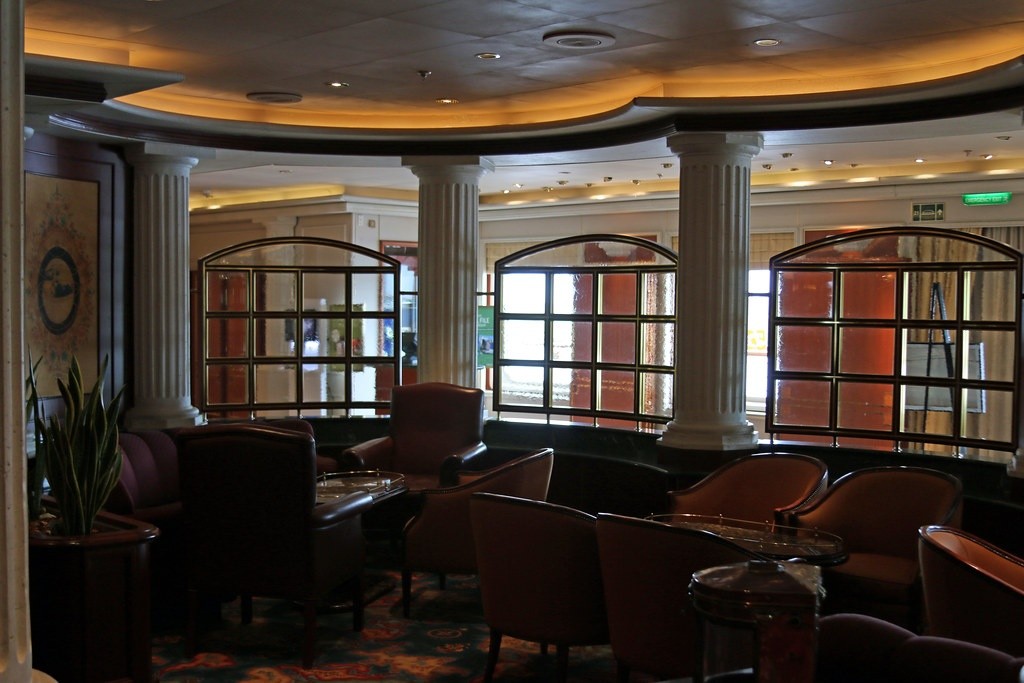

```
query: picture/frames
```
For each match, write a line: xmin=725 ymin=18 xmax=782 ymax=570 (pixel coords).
xmin=283 ymin=308 xmax=316 ymax=340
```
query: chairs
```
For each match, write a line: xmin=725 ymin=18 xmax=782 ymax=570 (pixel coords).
xmin=341 ymin=381 xmax=487 ymax=571
xmin=594 ymin=512 xmax=779 ymax=683
xmin=468 ymin=491 xmax=611 ymax=683
xmin=666 ymin=453 xmax=828 ymax=534
xmin=918 ymin=524 xmax=1024 ymax=657
xmin=401 ymin=448 xmax=553 ymax=618
xmin=793 ymin=467 xmax=962 ymax=620
xmin=177 ymin=424 xmax=371 ymax=669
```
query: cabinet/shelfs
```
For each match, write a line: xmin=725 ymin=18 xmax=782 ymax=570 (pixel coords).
xmin=376 ymin=368 xmax=418 ymax=413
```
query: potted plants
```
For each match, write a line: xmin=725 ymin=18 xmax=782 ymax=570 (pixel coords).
xmin=23 ymin=343 xmax=158 ymax=683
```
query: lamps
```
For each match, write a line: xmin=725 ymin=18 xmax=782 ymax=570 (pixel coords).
xmin=246 ymin=86 xmax=302 ymax=102
xmin=542 ymin=33 xmax=611 ymax=51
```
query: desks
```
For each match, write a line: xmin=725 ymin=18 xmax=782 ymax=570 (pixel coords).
xmin=646 ymin=512 xmax=848 ymax=592
xmin=313 ymin=472 xmax=408 ymax=556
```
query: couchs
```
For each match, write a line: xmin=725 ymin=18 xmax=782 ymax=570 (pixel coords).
xmin=101 ymin=418 xmax=336 ymax=524
xmin=813 ymin=613 xmax=1024 ymax=683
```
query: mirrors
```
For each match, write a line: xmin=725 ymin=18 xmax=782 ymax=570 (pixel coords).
xmin=380 ymin=241 xmax=419 ymax=362
